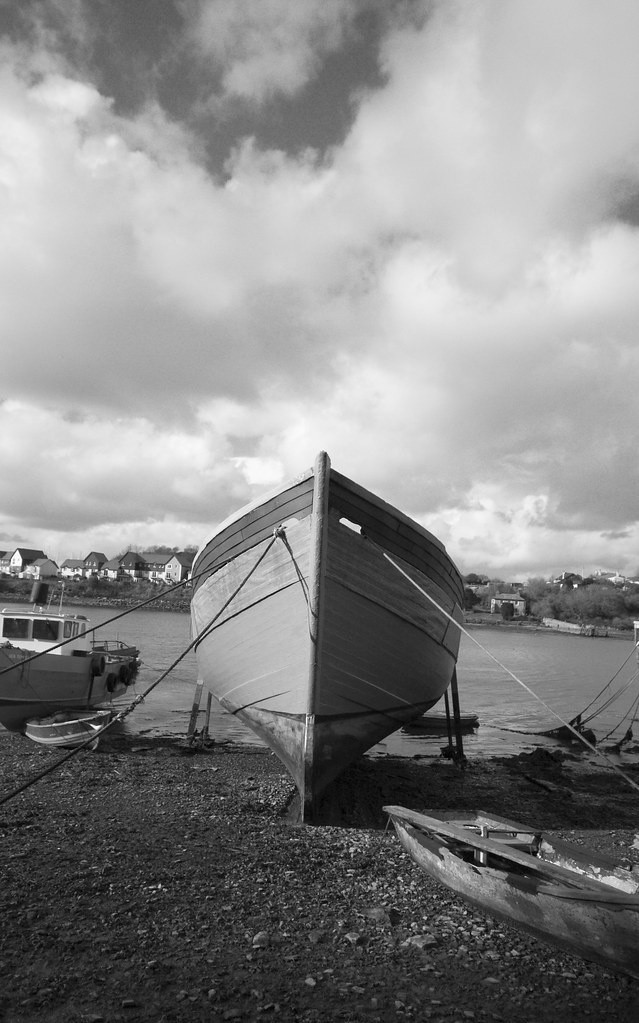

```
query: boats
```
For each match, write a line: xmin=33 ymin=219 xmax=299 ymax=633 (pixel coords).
xmin=0 ymin=581 xmax=141 ymax=731
xmin=402 ymin=713 xmax=480 ymax=732
xmin=389 ymin=806 xmax=639 ymax=982
xmin=25 ymin=709 xmax=111 ymax=750
xmin=189 ymin=452 xmax=465 ymax=822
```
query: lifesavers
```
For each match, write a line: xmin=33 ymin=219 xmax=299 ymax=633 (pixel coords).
xmin=120 ymin=662 xmax=135 ymax=687
xmin=91 ymin=653 xmax=106 ymax=677
xmin=107 ymin=673 xmax=118 ymax=692
